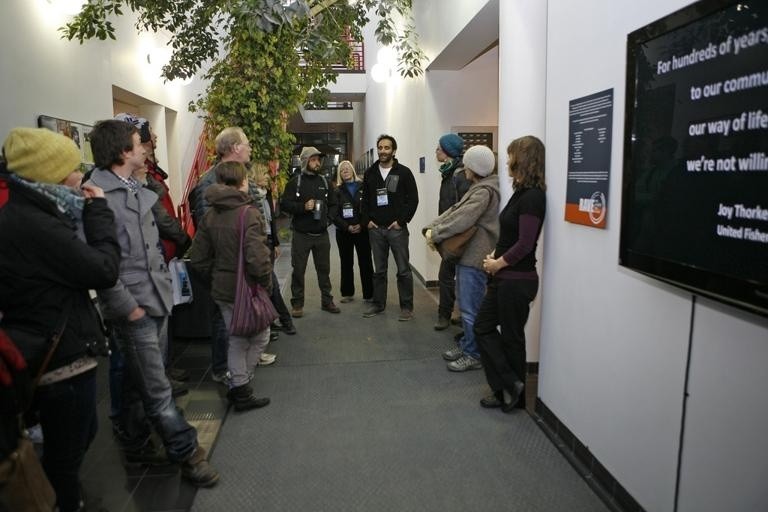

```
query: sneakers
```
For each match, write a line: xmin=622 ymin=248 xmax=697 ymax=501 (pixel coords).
xmin=213 ymin=354 xmax=277 ymax=411
xmin=321 ymin=303 xmax=339 ymax=313
xmin=363 ymin=305 xmax=385 ymax=317
xmin=125 ymin=443 xmax=219 ymax=487
xmin=399 ymin=309 xmax=414 ymax=321
xmin=481 ymin=379 xmax=524 ymax=412
xmin=434 ymin=316 xmax=463 ymax=330
xmin=442 ymin=346 xmax=482 ymax=371
xmin=280 ymin=308 xmax=302 ymax=335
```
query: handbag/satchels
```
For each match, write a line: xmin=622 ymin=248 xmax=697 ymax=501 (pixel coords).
xmin=435 ymin=226 xmax=477 ymax=262
xmin=229 ymin=276 xmax=279 ymax=336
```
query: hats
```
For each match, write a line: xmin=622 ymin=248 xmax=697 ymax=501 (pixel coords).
xmin=463 ymin=145 xmax=495 ymax=177
xmin=439 ymin=134 xmax=464 ymax=157
xmin=115 ymin=112 xmax=150 ymax=143
xmin=5 ymin=127 xmax=82 ymax=184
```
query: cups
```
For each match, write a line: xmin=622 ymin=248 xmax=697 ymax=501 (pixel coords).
xmin=311 ymin=200 xmax=322 ymax=220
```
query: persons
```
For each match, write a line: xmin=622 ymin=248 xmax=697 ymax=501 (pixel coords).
xmin=470 ymin=133 xmax=546 ymax=414
xmin=329 ymin=159 xmax=375 ymax=307
xmin=419 ymin=144 xmax=504 ymax=371
xmin=71 ymin=129 xmax=80 ymax=149
xmin=81 ymin=114 xmax=192 ymax=415
xmin=277 ymin=142 xmax=342 ymax=318
xmin=83 ymin=119 xmax=220 ymax=487
xmin=432 ymin=131 xmax=475 ymax=332
xmin=360 ymin=133 xmax=419 ymax=324
xmin=186 ymin=127 xmax=297 ymax=413
xmin=1 ymin=125 xmax=121 ymax=512
